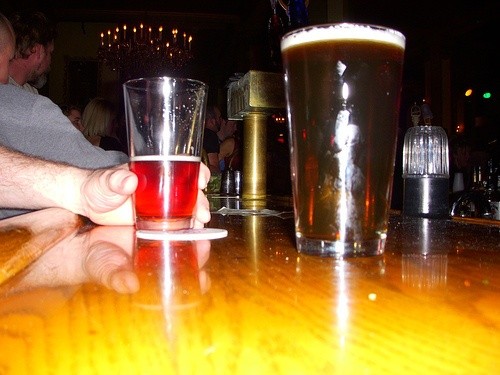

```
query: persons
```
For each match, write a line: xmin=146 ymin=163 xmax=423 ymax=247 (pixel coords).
xmin=0 ymin=10 xmax=238 ymax=229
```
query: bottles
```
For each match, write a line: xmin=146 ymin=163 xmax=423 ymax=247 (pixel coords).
xmin=220 ymin=168 xmax=236 ymax=196
xmin=234 ymin=171 xmax=242 ymax=194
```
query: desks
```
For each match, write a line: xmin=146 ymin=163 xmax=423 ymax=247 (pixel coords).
xmin=0 ymin=192 xmax=500 ymax=374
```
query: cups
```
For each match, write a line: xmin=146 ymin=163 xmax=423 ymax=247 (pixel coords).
xmin=402 ymin=125 xmax=450 ymax=218
xmin=280 ymin=24 xmax=406 ymax=256
xmin=123 ymin=78 xmax=207 ymax=231
